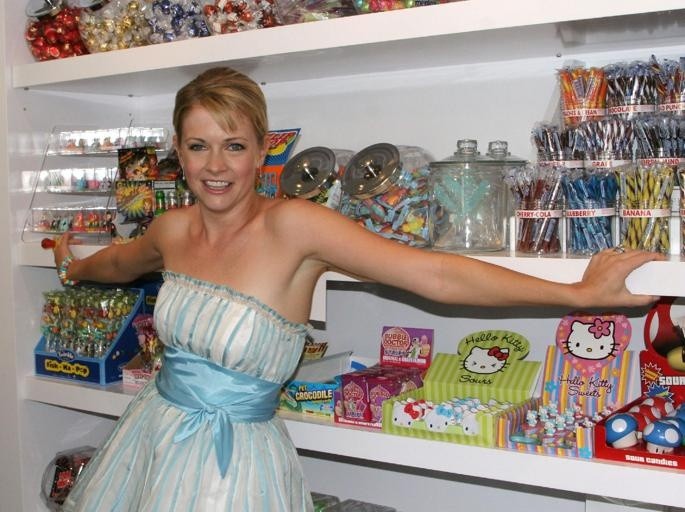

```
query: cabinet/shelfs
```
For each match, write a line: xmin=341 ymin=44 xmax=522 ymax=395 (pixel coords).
xmin=2 ymin=0 xmax=685 ymax=511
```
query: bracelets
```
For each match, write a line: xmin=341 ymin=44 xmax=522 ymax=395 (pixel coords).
xmin=57 ymin=255 xmax=81 ymax=288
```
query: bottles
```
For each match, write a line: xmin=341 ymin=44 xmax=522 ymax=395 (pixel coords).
xmin=426 ymin=136 xmax=534 ymax=252
xmin=333 ymin=142 xmax=457 ymax=252
xmin=276 ymin=145 xmax=354 ymax=216
xmin=23 ymin=0 xmax=449 ymax=62
xmin=151 ymin=190 xmax=194 ymax=222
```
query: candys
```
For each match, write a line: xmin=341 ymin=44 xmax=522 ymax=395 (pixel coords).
xmin=22 ymin=0 xmax=467 ymax=63
xmin=513 ymin=55 xmax=685 ymax=257
xmin=40 ymin=284 xmax=139 ymax=360
xmin=337 ymin=164 xmax=446 ymax=249
xmin=131 ymin=313 xmax=161 ymax=369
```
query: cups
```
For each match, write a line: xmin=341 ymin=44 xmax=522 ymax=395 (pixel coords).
xmin=514 ymin=94 xmax=684 ymax=263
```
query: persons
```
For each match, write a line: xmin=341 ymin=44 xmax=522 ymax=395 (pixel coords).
xmin=51 ymin=64 xmax=672 ymax=510
